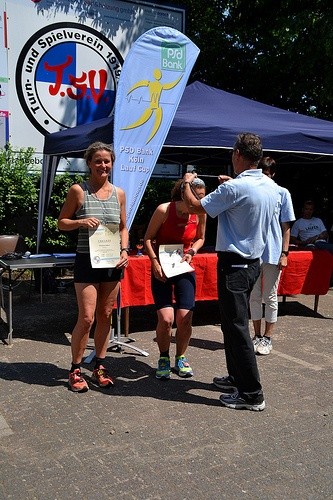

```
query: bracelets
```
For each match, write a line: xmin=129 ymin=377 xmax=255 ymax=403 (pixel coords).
xmin=186 ymin=248 xmax=197 ymax=256
xmin=150 ymin=257 xmax=158 ymax=260
xmin=183 ymin=182 xmax=191 ymax=184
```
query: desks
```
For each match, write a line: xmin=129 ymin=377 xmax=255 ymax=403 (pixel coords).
xmin=113 ymin=248 xmax=333 ymax=337
xmin=1 ymin=257 xmax=121 ymax=345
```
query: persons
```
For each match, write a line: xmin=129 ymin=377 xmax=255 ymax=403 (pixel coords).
xmin=290 ymin=200 xmax=333 ymax=254
xmin=184 ymin=133 xmax=279 ymax=410
xmin=144 ymin=177 xmax=206 ymax=380
xmin=250 ymin=157 xmax=296 ymax=355
xmin=58 ymin=142 xmax=128 ymax=392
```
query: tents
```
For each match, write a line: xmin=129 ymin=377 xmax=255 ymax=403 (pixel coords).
xmin=36 ymin=80 xmax=333 ymax=255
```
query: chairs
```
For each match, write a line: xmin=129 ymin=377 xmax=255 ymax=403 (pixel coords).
xmin=0 ymin=235 xmax=33 ymax=302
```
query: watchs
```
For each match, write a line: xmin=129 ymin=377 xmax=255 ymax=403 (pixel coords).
xmin=281 ymin=250 xmax=288 ymax=257
xmin=119 ymin=248 xmax=129 ymax=255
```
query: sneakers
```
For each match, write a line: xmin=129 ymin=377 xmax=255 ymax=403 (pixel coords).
xmin=68 ymin=368 xmax=90 ymax=393
xmin=257 ymin=337 xmax=273 ymax=355
xmin=213 ymin=375 xmax=238 ymax=389
xmin=174 ymin=354 xmax=193 ymax=378
xmin=252 ymin=336 xmax=263 ymax=352
xmin=220 ymin=391 xmax=265 ymax=411
xmin=91 ymin=365 xmax=114 ymax=386
xmin=155 ymin=356 xmax=171 ymax=379
